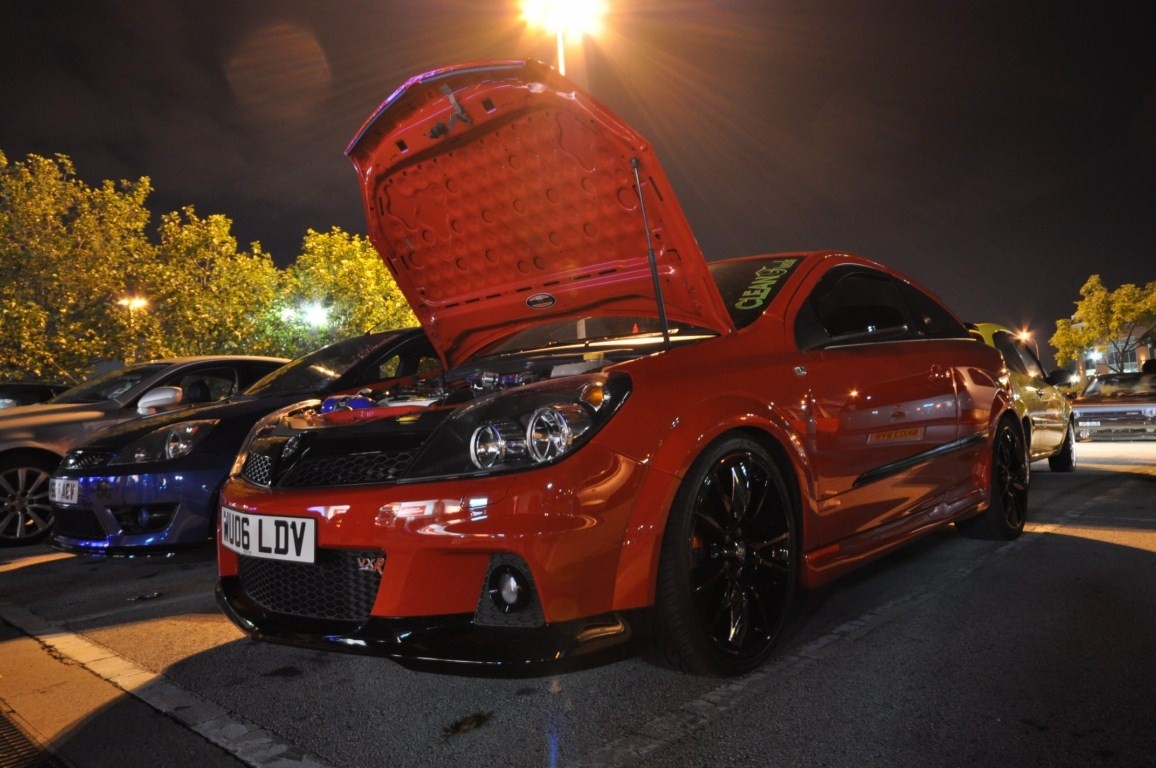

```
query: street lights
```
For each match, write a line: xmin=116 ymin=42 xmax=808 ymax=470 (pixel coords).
xmin=523 ymin=0 xmax=611 ymax=78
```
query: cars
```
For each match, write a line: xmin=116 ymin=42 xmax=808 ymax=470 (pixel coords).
xmin=213 ymin=54 xmax=1031 ymax=678
xmin=0 ymin=354 xmax=345 ymax=547
xmin=1070 ymin=371 xmax=1156 ymax=443
xmin=962 ymin=321 xmax=1077 ymax=474
xmin=42 ymin=324 xmax=445 ymax=561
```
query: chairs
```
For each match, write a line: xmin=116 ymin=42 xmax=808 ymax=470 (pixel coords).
xmin=187 ymin=380 xmax=211 ymax=404
